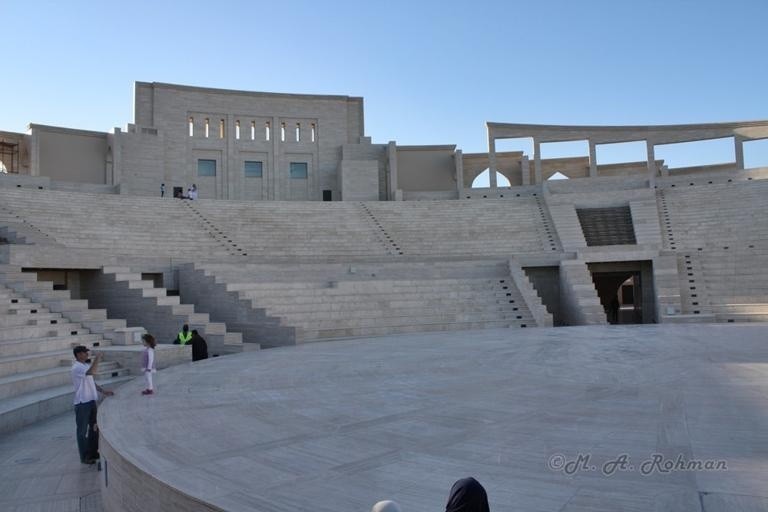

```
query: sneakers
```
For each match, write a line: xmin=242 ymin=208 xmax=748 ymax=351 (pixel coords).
xmin=80 ymin=451 xmax=101 ymax=464
xmin=142 ymin=389 xmax=154 ymax=395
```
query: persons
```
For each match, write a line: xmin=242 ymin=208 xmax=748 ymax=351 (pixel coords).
xmin=184 ymin=330 xmax=209 ymax=362
xmin=177 ymin=190 xmax=186 ymax=199
xmin=187 ymin=188 xmax=191 ymax=198
xmin=445 ymin=476 xmax=489 ymax=511
xmin=172 ymin=324 xmax=191 ymax=345
xmin=141 ymin=334 xmax=155 ymax=394
xmin=160 ymin=183 xmax=165 ymax=197
xmin=70 ymin=344 xmax=117 ymax=467
xmin=190 ymin=183 xmax=197 ymax=200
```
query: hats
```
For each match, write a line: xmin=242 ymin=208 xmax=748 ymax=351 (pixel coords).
xmin=73 ymin=345 xmax=90 ymax=354
